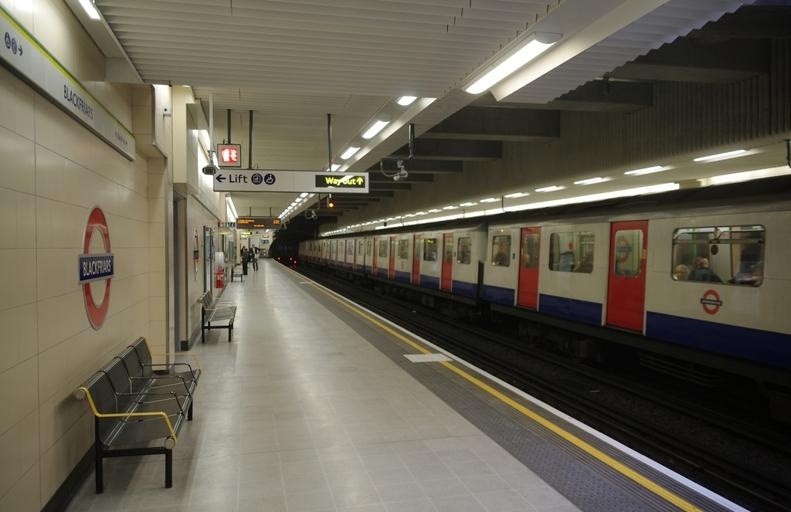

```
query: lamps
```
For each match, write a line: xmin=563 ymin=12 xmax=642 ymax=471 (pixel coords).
xmin=395 ymin=96 xmax=418 ymax=105
xmin=318 ymin=194 xmax=502 ymax=236
xmin=505 ymin=177 xmax=679 ymax=212
xmin=279 ymin=164 xmax=340 ymax=223
xmin=340 ymin=146 xmax=360 ymax=159
xmin=695 ymin=150 xmax=749 ymax=165
xmin=461 ymin=31 xmax=563 ymax=95
xmin=362 ymin=119 xmax=389 ymax=139
xmin=627 ymin=165 xmax=671 ymax=178
xmin=700 ymin=165 xmax=790 ymax=187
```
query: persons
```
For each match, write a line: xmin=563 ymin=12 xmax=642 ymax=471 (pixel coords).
xmin=574 ymin=253 xmax=594 ymax=273
xmin=754 ymin=260 xmax=764 ymax=287
xmin=524 ymin=252 xmax=534 ymax=269
xmin=494 ymin=251 xmax=509 ymax=267
xmin=674 ymin=264 xmax=690 ymax=280
xmin=691 ymin=257 xmax=724 ymax=283
xmin=240 ymin=245 xmax=245 ymax=257
xmin=250 ymin=244 xmax=260 ymax=272
xmin=242 ymin=248 xmax=249 ymax=275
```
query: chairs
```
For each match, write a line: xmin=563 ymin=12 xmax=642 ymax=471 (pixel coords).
xmin=75 ymin=263 xmax=244 ymax=494
